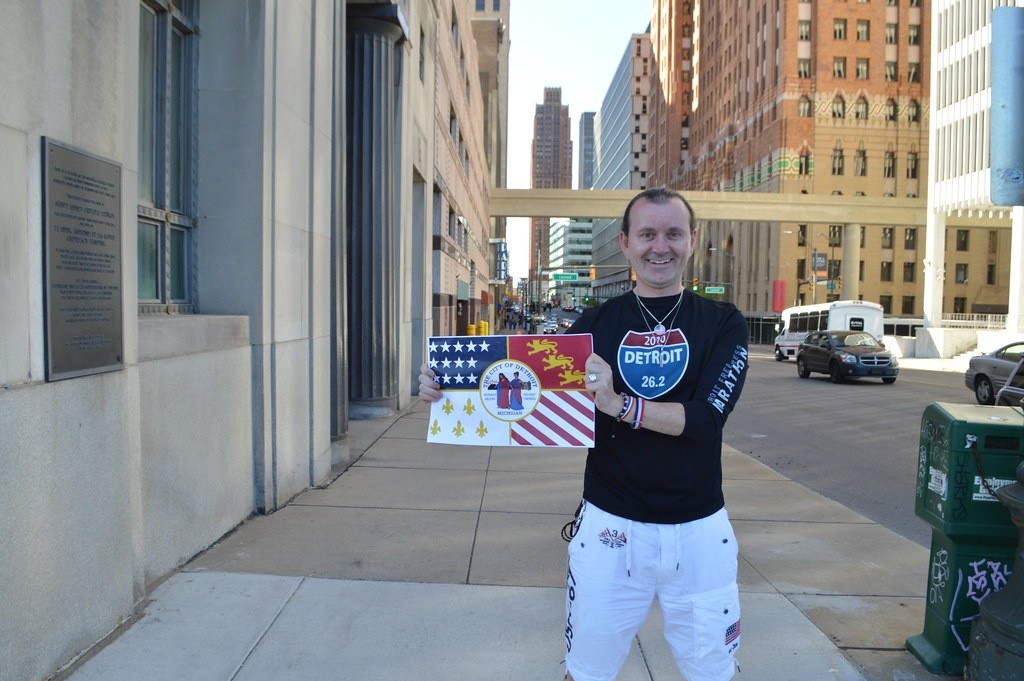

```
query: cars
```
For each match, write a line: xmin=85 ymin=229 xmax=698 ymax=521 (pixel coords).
xmin=964 ymin=341 xmax=1024 ymax=405
xmin=561 ymin=318 xmax=575 ymax=328
xmin=796 ymin=330 xmax=900 ymax=384
xmin=534 ymin=313 xmax=560 ymax=334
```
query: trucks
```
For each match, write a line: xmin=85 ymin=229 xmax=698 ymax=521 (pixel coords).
xmin=773 ymin=300 xmax=884 ymax=362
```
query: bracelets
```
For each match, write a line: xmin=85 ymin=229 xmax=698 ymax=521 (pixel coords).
xmin=630 ymin=397 xmax=645 ymax=430
xmin=616 ymin=391 xmax=634 ymax=423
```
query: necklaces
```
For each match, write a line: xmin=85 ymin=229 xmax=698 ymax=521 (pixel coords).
xmin=636 ymin=289 xmax=683 ymax=368
xmin=633 ymin=289 xmax=683 ymax=336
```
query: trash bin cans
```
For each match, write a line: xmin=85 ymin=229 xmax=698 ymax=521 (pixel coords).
xmin=905 ymin=401 xmax=1024 ymax=675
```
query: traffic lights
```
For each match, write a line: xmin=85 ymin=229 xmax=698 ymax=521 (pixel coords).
xmin=692 ymin=278 xmax=699 ymax=291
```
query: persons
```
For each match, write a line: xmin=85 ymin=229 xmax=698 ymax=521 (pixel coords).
xmin=417 ymin=187 xmax=749 ymax=681
xmin=505 ymin=313 xmax=522 ymax=331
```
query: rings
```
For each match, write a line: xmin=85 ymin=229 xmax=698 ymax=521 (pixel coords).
xmin=589 ymin=373 xmax=597 ymax=383
